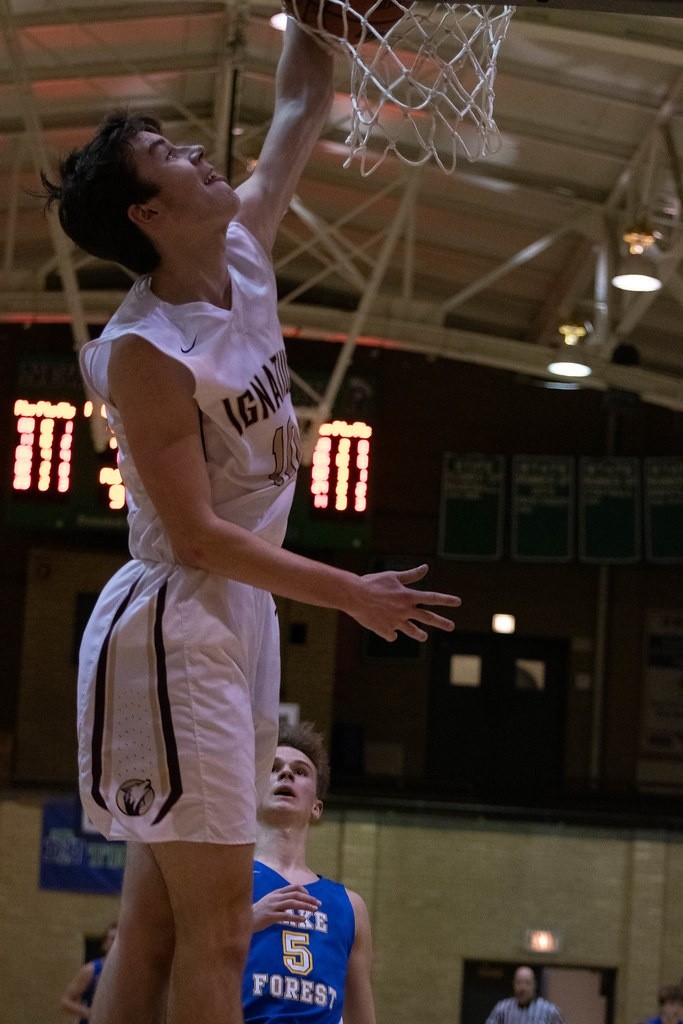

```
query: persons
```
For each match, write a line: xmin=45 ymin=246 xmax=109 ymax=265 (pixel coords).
xmin=485 ymin=966 xmax=565 ymax=1024
xmin=640 ymin=984 xmax=683 ymax=1024
xmin=62 ymin=922 xmax=117 ymax=1023
xmin=36 ymin=19 xmax=461 ymax=1024
xmin=242 ymin=723 xmax=377 ymax=1024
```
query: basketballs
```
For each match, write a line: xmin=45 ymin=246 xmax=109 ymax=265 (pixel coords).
xmin=286 ymin=0 xmax=412 ymax=46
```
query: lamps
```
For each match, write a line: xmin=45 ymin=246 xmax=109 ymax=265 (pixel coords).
xmin=623 ymin=205 xmax=655 ymax=255
xmin=558 ymin=304 xmax=594 ymax=346
xmin=610 ymin=254 xmax=662 ymax=292
xmin=547 ymin=341 xmax=592 ymax=378
